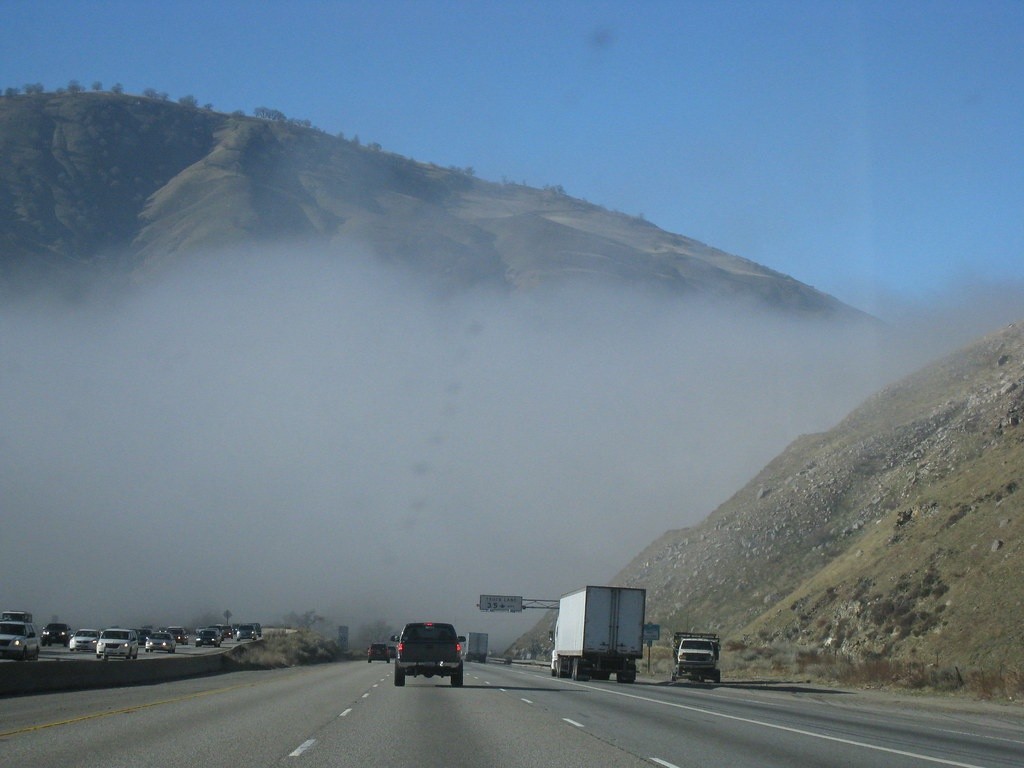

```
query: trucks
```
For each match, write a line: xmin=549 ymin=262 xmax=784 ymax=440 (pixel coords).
xmin=548 ymin=586 xmax=646 ymax=683
xmin=466 ymin=632 xmax=488 ymax=664
xmin=671 ymin=632 xmax=721 ymax=683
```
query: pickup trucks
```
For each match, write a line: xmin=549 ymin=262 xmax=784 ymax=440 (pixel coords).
xmin=390 ymin=622 xmax=466 ymax=688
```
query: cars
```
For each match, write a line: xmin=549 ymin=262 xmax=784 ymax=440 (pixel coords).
xmin=167 ymin=627 xmax=189 ymax=645
xmin=96 ymin=629 xmax=138 ymax=659
xmin=367 ymin=643 xmax=390 ymax=663
xmin=40 ymin=623 xmax=73 ymax=646
xmin=388 ymin=646 xmax=396 ymax=658
xmin=146 ymin=633 xmax=176 ymax=653
xmin=131 ymin=628 xmax=153 ymax=645
xmin=69 ymin=629 xmax=100 ymax=651
xmin=195 ymin=630 xmax=222 ymax=647
xmin=249 ymin=623 xmax=263 ymax=637
xmin=234 ymin=625 xmax=257 ymax=641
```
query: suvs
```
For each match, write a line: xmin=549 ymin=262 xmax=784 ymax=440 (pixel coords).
xmin=0 ymin=619 xmax=41 ymax=661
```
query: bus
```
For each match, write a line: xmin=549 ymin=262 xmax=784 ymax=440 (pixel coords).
xmin=210 ymin=625 xmax=232 ymax=638
xmin=2 ymin=610 xmax=33 ymax=623
